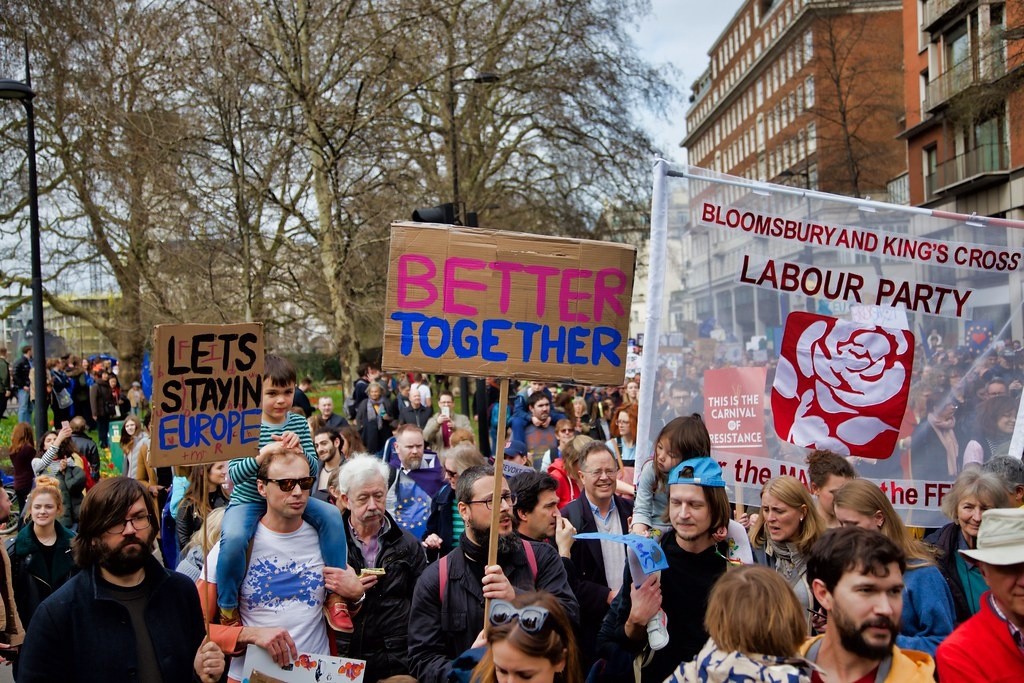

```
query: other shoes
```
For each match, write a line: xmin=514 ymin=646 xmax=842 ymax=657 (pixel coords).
xmin=323 ymin=598 xmax=354 ymax=634
xmin=220 ymin=610 xmax=246 ymax=657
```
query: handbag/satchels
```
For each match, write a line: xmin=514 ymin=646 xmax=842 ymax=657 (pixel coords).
xmin=55 ymin=387 xmax=74 ymax=409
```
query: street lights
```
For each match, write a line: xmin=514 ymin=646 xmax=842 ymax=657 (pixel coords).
xmin=688 ymin=229 xmax=712 ymax=319
xmin=776 ymin=169 xmax=813 ymax=266
xmin=0 ymin=76 xmax=50 ymax=458
xmin=447 ymin=68 xmax=502 ymax=225
xmin=668 ymin=235 xmax=685 ymax=287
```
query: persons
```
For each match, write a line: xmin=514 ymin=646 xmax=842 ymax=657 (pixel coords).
xmin=0 ymin=334 xmax=1024 ymax=683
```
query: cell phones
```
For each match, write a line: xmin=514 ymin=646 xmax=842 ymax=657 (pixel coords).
xmin=807 ymin=608 xmax=827 ymax=619
xmin=61 ymin=420 xmax=70 ymax=429
xmin=442 ymin=407 xmax=450 ymax=418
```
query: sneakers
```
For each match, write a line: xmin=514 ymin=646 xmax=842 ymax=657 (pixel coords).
xmin=647 ymin=608 xmax=670 ymax=650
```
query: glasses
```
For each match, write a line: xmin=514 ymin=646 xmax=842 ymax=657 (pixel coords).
xmin=559 ymin=428 xmax=575 ymax=433
xmin=263 ymin=476 xmax=316 ymax=492
xmin=444 ymin=466 xmax=457 ymax=478
xmin=582 ymin=470 xmax=617 ymax=477
xmin=107 ymin=514 xmax=152 ymax=534
xmin=464 ymin=493 xmax=518 ymax=510
xmin=987 ymin=392 xmax=1005 ymax=398
xmin=489 ymin=599 xmax=569 ymax=645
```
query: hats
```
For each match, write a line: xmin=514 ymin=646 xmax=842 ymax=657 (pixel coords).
xmin=958 ymin=508 xmax=1024 ymax=565
xmin=1000 ymin=347 xmax=1017 ymax=358
xmin=668 ymin=457 xmax=725 ymax=487
xmin=504 ymin=440 xmax=527 ymax=457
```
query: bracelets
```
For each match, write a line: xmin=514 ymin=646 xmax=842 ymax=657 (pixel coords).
xmin=347 ymin=592 xmax=366 ymax=612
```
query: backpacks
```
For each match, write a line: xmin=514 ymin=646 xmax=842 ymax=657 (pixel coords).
xmin=344 ymin=380 xmax=368 ymax=420
